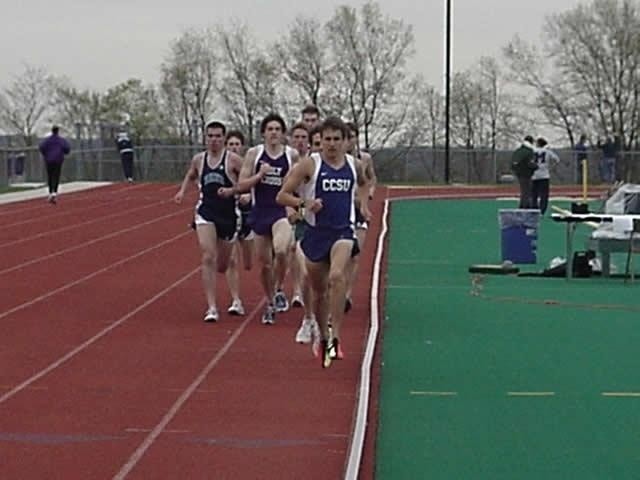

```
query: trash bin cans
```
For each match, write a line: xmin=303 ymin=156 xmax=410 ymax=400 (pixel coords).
xmin=497 ymin=208 xmax=541 ymax=264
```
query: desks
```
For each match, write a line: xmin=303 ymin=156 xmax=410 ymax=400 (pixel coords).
xmin=552 ymin=211 xmax=640 ymax=280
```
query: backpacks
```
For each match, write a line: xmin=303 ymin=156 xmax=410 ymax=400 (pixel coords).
xmin=512 ymin=148 xmax=530 ymax=175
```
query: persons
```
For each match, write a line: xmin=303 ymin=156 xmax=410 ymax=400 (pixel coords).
xmin=597 ymin=138 xmax=620 ymax=185
xmin=575 ymin=134 xmax=586 ymax=184
xmin=38 ymin=125 xmax=72 ymax=202
xmin=511 ymin=136 xmax=537 ymax=208
xmin=114 ymin=126 xmax=135 ymax=184
xmin=521 ymin=139 xmax=560 ymax=215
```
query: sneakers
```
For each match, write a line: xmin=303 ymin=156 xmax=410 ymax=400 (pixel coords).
xmin=343 ymin=291 xmax=352 ymax=313
xmin=295 ymin=314 xmax=344 ymax=369
xmin=227 ymin=297 xmax=248 ymax=315
xmin=272 ymin=288 xmax=288 ymax=313
xmin=204 ymin=307 xmax=220 ymax=323
xmin=260 ymin=303 xmax=276 ymax=326
xmin=47 ymin=192 xmax=57 ymax=205
xmin=291 ymin=290 xmax=304 ymax=307
xmin=127 ymin=176 xmax=136 ymax=184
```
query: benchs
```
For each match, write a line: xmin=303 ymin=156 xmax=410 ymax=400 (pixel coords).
xmin=584 ymin=234 xmax=640 ymax=278
